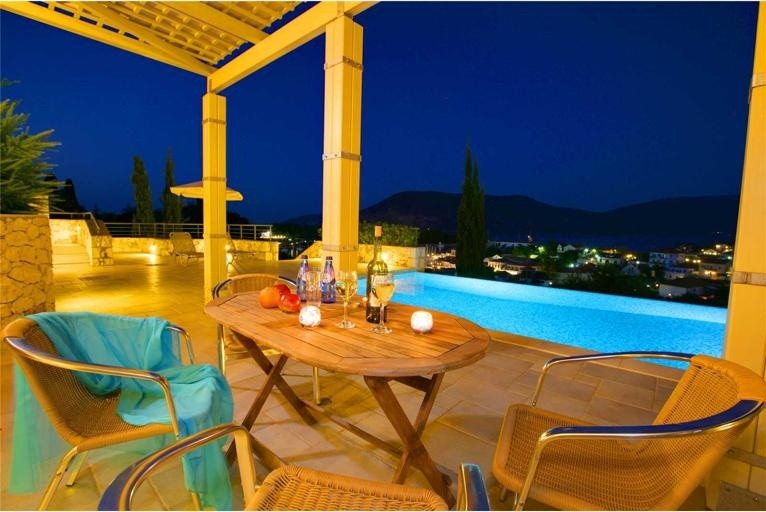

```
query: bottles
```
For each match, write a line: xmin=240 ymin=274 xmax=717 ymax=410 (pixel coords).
xmin=319 ymin=255 xmax=336 ymax=304
xmin=296 ymin=253 xmax=310 ymax=302
xmin=366 ymin=224 xmax=388 ymax=323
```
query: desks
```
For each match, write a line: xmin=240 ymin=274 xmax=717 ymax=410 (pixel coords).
xmin=191 ymin=249 xmax=255 ymax=275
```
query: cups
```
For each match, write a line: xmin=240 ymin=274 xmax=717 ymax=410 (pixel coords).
xmin=305 ymin=269 xmax=323 ymax=308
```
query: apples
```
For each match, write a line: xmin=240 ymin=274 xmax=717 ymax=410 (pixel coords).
xmin=278 ymin=294 xmax=301 ymax=312
xmin=272 ymin=284 xmax=290 ymax=297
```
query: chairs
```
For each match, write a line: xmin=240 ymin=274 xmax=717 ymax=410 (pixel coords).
xmin=491 ymin=350 xmax=766 ymax=511
xmin=99 ymin=422 xmax=490 ymax=511
xmin=170 ymin=232 xmax=198 ymax=265
xmin=211 ymin=273 xmax=322 ymax=407
xmin=1 ymin=312 xmax=234 ymax=511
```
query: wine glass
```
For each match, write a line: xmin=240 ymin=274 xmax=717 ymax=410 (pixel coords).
xmin=333 ymin=269 xmax=358 ymax=330
xmin=369 ymin=271 xmax=396 ymax=334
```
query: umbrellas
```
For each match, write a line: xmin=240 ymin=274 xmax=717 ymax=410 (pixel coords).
xmin=170 ymin=179 xmax=244 ymax=203
